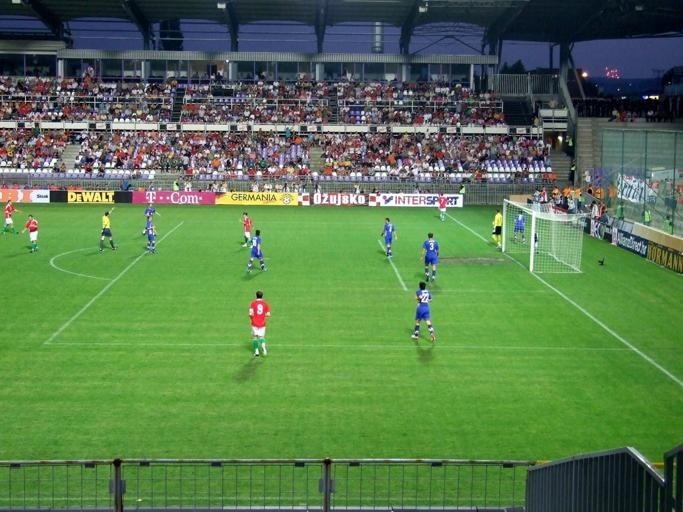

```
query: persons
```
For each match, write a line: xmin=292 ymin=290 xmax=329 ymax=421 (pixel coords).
xmin=240 ymin=212 xmax=254 ymax=249
xmin=411 ymin=282 xmax=436 ymax=344
xmin=142 ymin=223 xmax=158 ymax=254
xmin=1 ymin=69 xmax=682 ymax=234
xmin=246 ymin=229 xmax=267 ymax=272
xmin=99 ymin=207 xmax=118 ymax=251
xmin=21 ymin=214 xmax=39 ymax=254
xmin=380 ymin=217 xmax=397 ymax=258
xmin=513 ymin=210 xmax=527 ymax=244
xmin=420 ymin=232 xmax=439 ymax=284
xmin=248 ymin=291 xmax=271 ymax=358
xmin=491 ymin=208 xmax=503 ymax=249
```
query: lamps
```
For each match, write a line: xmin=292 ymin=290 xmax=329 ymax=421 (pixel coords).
xmin=418 ymin=1 xmax=431 ymax=17
xmin=216 ymin=1 xmax=228 ymax=12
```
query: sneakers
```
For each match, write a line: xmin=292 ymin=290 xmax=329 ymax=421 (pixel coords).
xmin=389 ymin=252 xmax=392 ymax=257
xmin=254 ymin=350 xmax=260 ymax=356
xmin=262 ymin=266 xmax=266 ymax=271
xmin=260 ymin=346 xmax=266 ymax=356
xmin=432 ymin=275 xmax=435 ymax=281
xmin=431 ymin=333 xmax=434 ymax=342
xmin=387 ymin=253 xmax=389 ymax=257
xmin=426 ymin=272 xmax=430 ymax=282
xmin=410 ymin=334 xmax=418 ymax=338
xmin=247 ymin=268 xmax=250 ymax=272
